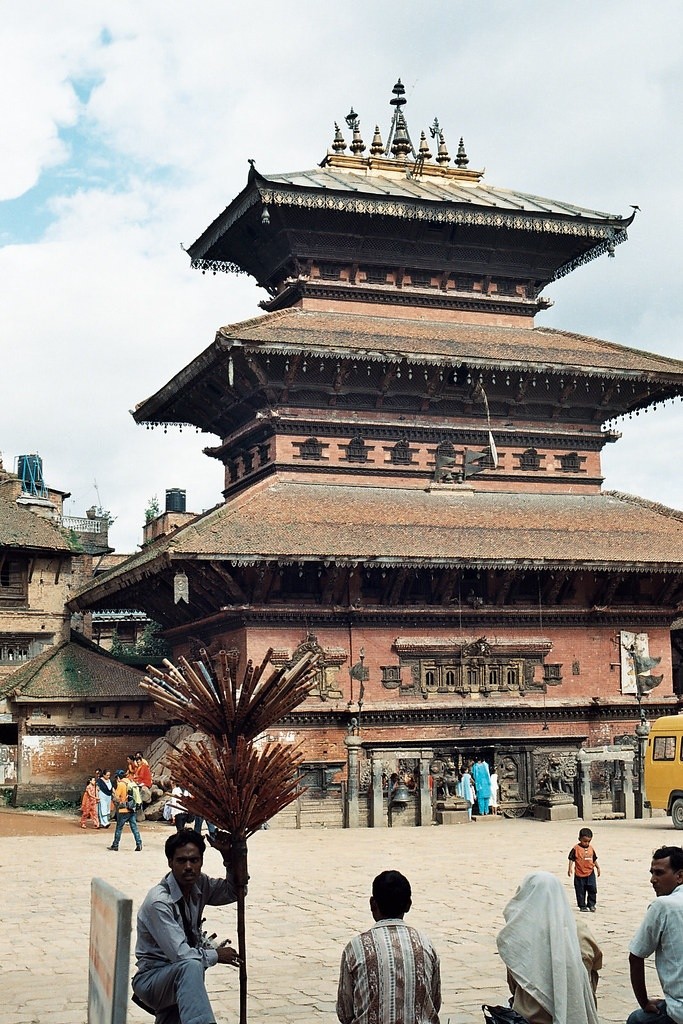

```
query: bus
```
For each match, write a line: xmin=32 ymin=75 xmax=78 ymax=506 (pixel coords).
xmin=642 ymin=714 xmax=682 ymax=830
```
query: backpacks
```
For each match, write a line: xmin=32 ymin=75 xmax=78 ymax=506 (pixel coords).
xmin=118 ymin=778 xmax=143 ymax=813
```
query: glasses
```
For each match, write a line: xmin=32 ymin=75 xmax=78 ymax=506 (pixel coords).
xmin=96 ymin=772 xmax=99 ymax=775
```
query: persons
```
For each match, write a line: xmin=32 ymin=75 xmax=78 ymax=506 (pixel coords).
xmin=105 ymin=770 xmax=144 ymax=851
xmin=336 ymin=870 xmax=441 ymax=1023
xmin=77 ymin=749 xmax=217 ymax=837
xmin=496 ymin=871 xmax=602 ymax=1023
xmin=624 ymin=846 xmax=683 ymax=1023
xmin=566 ymin=828 xmax=603 ymax=910
xmin=130 ymin=828 xmax=244 ymax=1023
xmin=458 ymin=756 xmax=499 ymax=817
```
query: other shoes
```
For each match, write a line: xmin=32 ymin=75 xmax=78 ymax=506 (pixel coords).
xmin=589 ymin=906 xmax=596 ymax=912
xmin=580 ymin=907 xmax=588 ymax=912
xmin=107 ymin=846 xmax=118 ymax=851
xmin=135 ymin=846 xmax=142 ymax=851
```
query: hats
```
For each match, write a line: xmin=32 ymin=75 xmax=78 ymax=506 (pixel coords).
xmin=113 ymin=769 xmax=124 ymax=778
